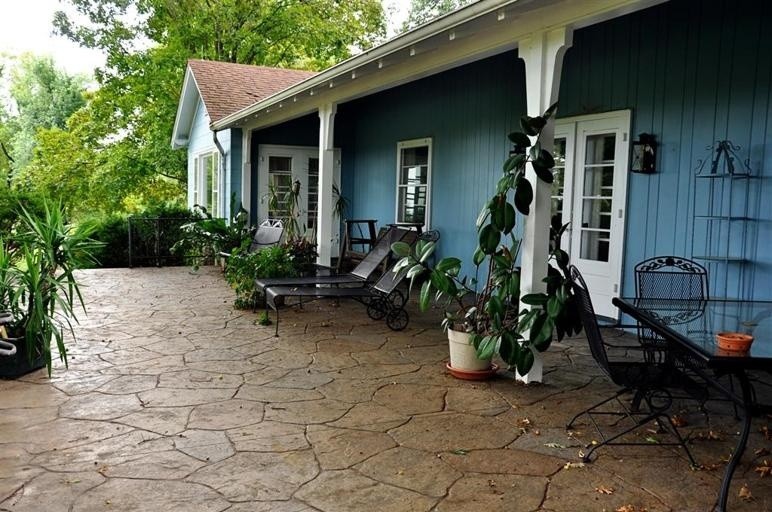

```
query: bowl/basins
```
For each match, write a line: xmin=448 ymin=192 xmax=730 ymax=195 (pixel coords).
xmin=716 ymin=332 xmax=754 ymax=352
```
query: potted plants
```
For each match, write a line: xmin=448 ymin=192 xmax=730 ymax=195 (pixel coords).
xmin=393 ymin=102 xmax=583 ymax=379
xmin=0 ymin=186 xmax=109 ymax=381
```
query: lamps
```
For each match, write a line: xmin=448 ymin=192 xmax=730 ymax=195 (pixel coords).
xmin=628 ymin=129 xmax=658 ymax=176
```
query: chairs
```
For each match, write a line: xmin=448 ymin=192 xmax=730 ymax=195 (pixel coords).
xmin=219 ymin=221 xmax=441 ymax=338
xmin=566 ymin=256 xmax=743 ymax=469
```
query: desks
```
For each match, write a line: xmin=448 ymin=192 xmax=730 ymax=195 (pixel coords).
xmin=613 ymin=296 xmax=772 ymax=512
